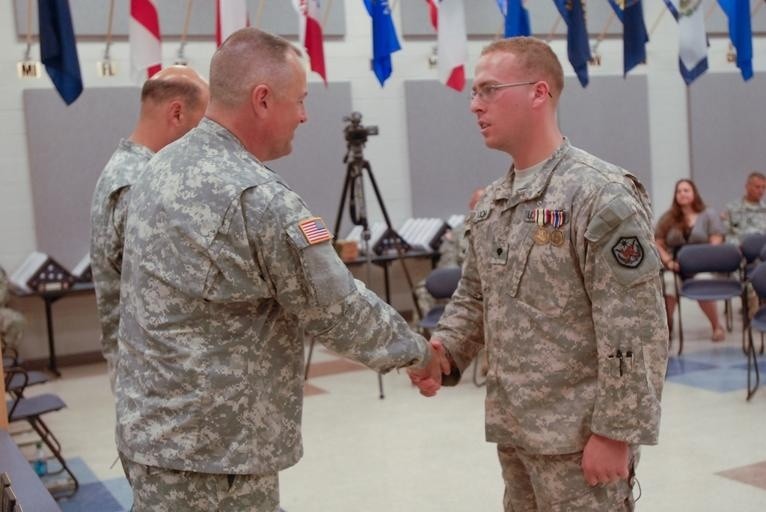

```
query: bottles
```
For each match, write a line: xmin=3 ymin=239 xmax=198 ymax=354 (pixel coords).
xmin=32 ymin=442 xmax=46 ymax=475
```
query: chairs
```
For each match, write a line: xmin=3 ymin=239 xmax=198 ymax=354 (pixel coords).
xmin=0 ymin=332 xmax=79 ymax=503
xmin=655 ymin=228 xmax=766 ymax=401
xmin=416 ymin=263 xmax=489 ymax=391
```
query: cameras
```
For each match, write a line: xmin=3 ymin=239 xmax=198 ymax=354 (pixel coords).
xmin=342 ymin=111 xmax=378 ymax=143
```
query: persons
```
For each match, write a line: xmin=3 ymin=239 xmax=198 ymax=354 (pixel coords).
xmin=114 ymin=26 xmax=444 ymax=512
xmin=411 ymin=189 xmax=486 ymax=334
xmin=653 ymin=176 xmax=726 ymax=346
xmin=92 ymin=64 xmax=210 ymax=393
xmin=722 ymin=171 xmax=766 ymax=324
xmin=408 ymin=36 xmax=670 ymax=512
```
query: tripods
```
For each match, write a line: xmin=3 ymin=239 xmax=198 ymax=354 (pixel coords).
xmin=304 ymin=143 xmax=432 ymax=400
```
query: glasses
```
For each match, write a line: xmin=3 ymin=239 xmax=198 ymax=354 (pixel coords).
xmin=470 ymin=80 xmax=554 ymax=103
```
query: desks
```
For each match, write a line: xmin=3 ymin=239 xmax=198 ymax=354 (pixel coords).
xmin=8 ymin=281 xmax=97 ymax=379
xmin=339 ymin=249 xmax=442 ymax=310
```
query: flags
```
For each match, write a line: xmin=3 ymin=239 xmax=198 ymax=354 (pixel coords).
xmin=293 ymin=0 xmax=326 ymax=81
xmin=665 ymin=0 xmax=709 ymax=84
xmin=498 ymin=1 xmax=530 ymax=38
xmin=215 ymin=0 xmax=250 ymax=48
xmin=718 ymin=0 xmax=753 ymax=80
xmin=364 ymin=0 xmax=401 ymax=86
xmin=428 ymin=0 xmax=468 ymax=91
xmin=130 ymin=1 xmax=162 ymax=84
xmin=554 ymin=1 xmax=590 ymax=87
xmin=38 ymin=0 xmax=84 ymax=104
xmin=609 ymin=0 xmax=650 ymax=73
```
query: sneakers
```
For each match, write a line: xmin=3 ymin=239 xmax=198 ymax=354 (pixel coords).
xmin=712 ymin=324 xmax=726 ymax=341
